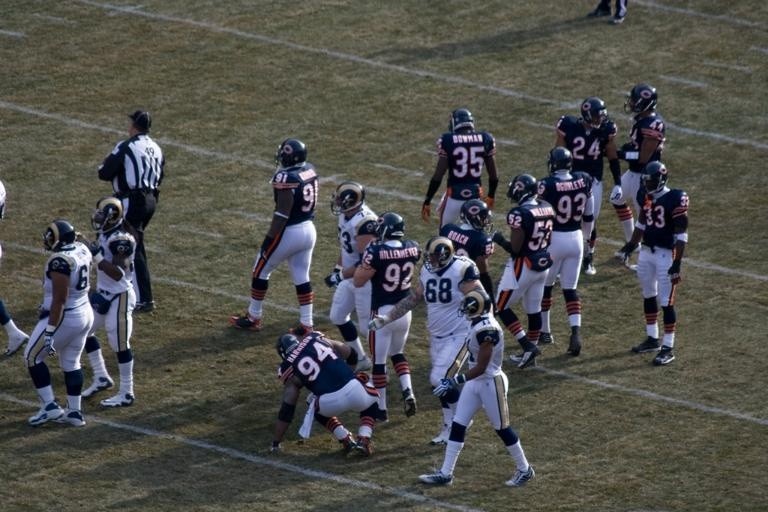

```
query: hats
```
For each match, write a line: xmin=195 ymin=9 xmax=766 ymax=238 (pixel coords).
xmin=127 ymin=110 xmax=151 ymax=131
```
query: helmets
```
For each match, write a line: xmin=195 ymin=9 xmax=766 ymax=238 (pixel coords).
xmin=460 ymin=199 xmax=492 ymax=230
xmin=276 ymin=333 xmax=301 ymax=359
xmin=448 ymin=109 xmax=474 ymax=132
xmin=581 ymin=97 xmax=609 ymax=128
xmin=43 ymin=219 xmax=75 ymax=251
xmin=276 ymin=138 xmax=306 ymax=170
xmin=423 ymin=236 xmax=454 ymax=273
xmin=624 ymin=83 xmax=657 ymax=114
xmin=376 ymin=212 xmax=407 ymax=242
xmin=546 ymin=145 xmax=574 ymax=174
xmin=641 ymin=161 xmax=668 ymax=195
xmin=457 ymin=291 xmax=491 ymax=321
xmin=506 ymin=173 xmax=538 ymax=205
xmin=90 ymin=197 xmax=124 ymax=233
xmin=330 ymin=182 xmax=365 ymax=216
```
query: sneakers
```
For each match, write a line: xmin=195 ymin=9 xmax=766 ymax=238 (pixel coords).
xmin=419 ymin=472 xmax=454 ymax=485
xmin=505 ymin=466 xmax=536 ymax=487
xmin=29 ymin=401 xmax=64 ymax=428
xmin=342 ymin=434 xmax=357 ymax=453
xmin=289 ymin=322 xmax=313 ymax=336
xmin=509 ymin=353 xmax=524 ymax=363
xmin=517 ymin=348 xmax=541 ymax=370
xmin=405 ymin=392 xmax=417 ymax=416
xmin=376 ymin=408 xmax=388 ymax=422
xmin=540 ymin=331 xmax=553 ymax=343
xmin=354 ymin=356 xmax=372 ymax=373
xmin=582 ymin=253 xmax=597 ymax=276
xmin=101 ymin=390 xmax=134 ymax=407
xmin=5 ymin=333 xmax=28 ymax=357
xmin=133 ymin=298 xmax=155 ymax=313
xmin=356 ymin=435 xmax=372 ymax=453
xmin=51 ymin=406 xmax=86 ymax=427
xmin=632 ymin=336 xmax=662 ymax=354
xmin=568 ymin=334 xmax=581 ymax=356
xmin=654 ymin=346 xmax=675 ymax=366
xmin=80 ymin=376 xmax=115 ymax=398
xmin=429 ymin=431 xmax=450 ymax=445
xmin=230 ymin=313 xmax=262 ymax=331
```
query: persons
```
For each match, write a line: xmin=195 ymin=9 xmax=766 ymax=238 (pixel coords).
xmin=24 ymin=219 xmax=93 ymax=427
xmin=534 ymin=148 xmax=595 ymax=354
xmin=325 ymin=181 xmax=382 ymax=376
xmin=230 ymin=139 xmax=319 ymax=334
xmin=555 ymin=98 xmax=622 ymax=273
xmin=494 ymin=175 xmax=554 ymax=369
xmin=353 ymin=211 xmax=415 ymax=422
xmin=75 ymin=198 xmax=138 ymax=405
xmin=367 ymin=238 xmax=493 ymax=444
xmin=421 ymin=108 xmax=499 ymax=231
xmin=99 ymin=112 xmax=165 ymax=311
xmin=441 ymin=202 xmax=495 ymax=313
xmin=588 ymin=1 xmax=627 ymax=25
xmin=419 ymin=290 xmax=533 ymax=486
xmin=619 ymin=162 xmax=688 ymax=363
xmin=0 ymin=180 xmax=30 ymax=356
xmin=612 ymin=86 xmax=665 ymax=270
xmin=272 ymin=332 xmax=381 ymax=457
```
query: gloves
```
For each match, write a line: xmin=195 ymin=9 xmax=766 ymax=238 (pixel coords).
xmin=492 ymin=232 xmax=506 ymax=247
xmin=432 ymin=383 xmax=448 ymax=397
xmin=331 ymin=267 xmax=343 ymax=276
xmin=668 ymin=261 xmax=681 ymax=284
xmin=422 ymin=200 xmax=432 ymax=220
xmin=615 ymin=243 xmax=637 ymax=260
xmin=89 ymin=240 xmax=104 ymax=261
xmin=42 ymin=329 xmax=56 ymax=358
xmin=440 ymin=376 xmax=466 ymax=390
xmin=324 ymin=272 xmax=345 ymax=288
xmin=367 ymin=314 xmax=393 ymax=332
